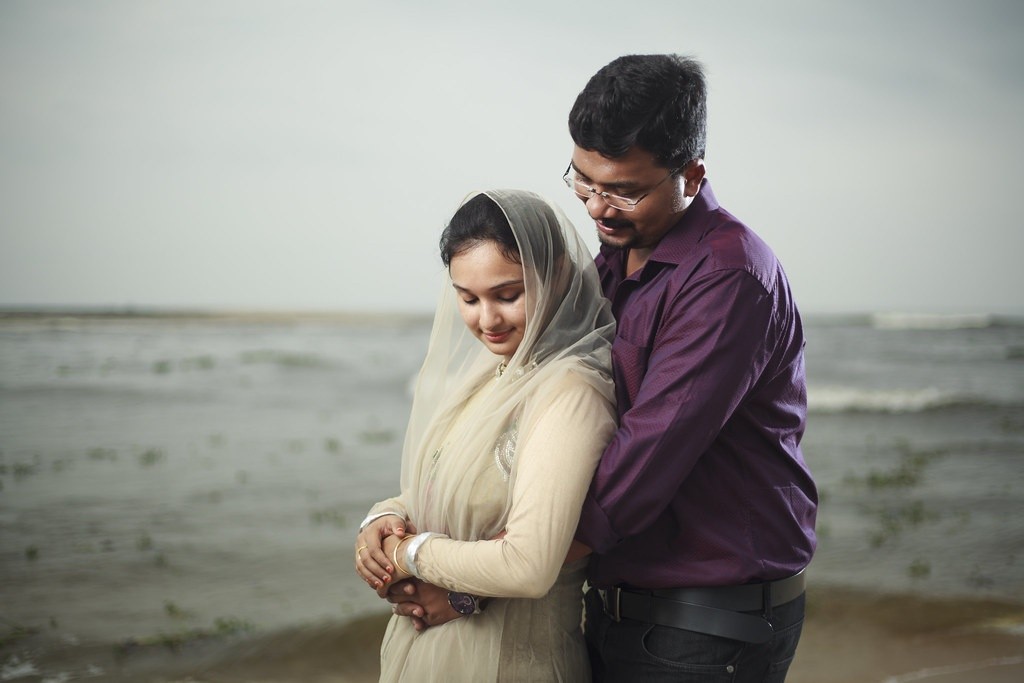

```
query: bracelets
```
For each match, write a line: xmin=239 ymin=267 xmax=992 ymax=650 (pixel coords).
xmin=394 ymin=533 xmax=418 ymax=577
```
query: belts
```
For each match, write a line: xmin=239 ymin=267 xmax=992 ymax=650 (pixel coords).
xmin=596 ymin=565 xmax=807 ymax=644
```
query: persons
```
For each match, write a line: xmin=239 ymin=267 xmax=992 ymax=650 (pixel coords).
xmin=354 ymin=190 xmax=616 ymax=683
xmin=355 ymin=56 xmax=817 ymax=682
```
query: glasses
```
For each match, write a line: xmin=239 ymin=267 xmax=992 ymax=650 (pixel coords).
xmin=562 ymin=158 xmax=692 ymax=212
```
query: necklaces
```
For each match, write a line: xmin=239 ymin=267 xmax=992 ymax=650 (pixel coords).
xmin=493 ymin=358 xmax=540 ymax=377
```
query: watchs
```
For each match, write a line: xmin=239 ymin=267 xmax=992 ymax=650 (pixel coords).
xmin=445 ymin=589 xmax=481 ymax=618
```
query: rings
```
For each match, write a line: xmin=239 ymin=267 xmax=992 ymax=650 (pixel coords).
xmin=358 ymin=545 xmax=368 ymax=555
